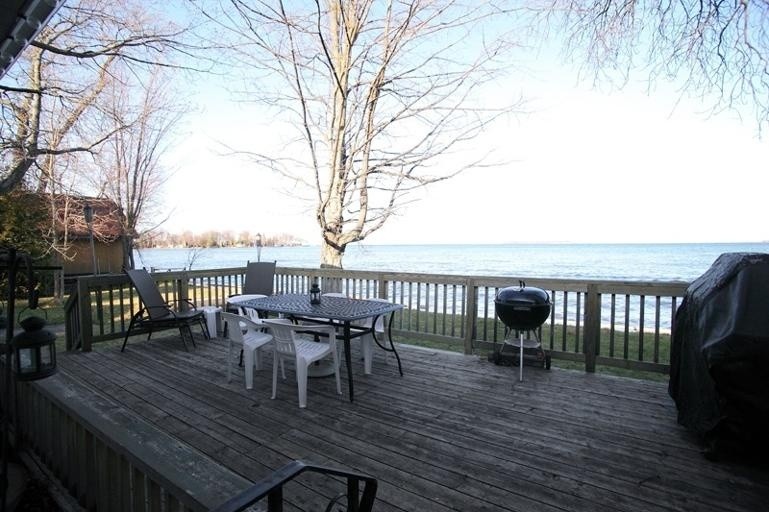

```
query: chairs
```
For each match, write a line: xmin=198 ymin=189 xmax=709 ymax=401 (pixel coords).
xmin=220 ymin=292 xmax=387 ymax=408
xmin=223 ymin=260 xmax=276 ymax=338
xmin=121 ymin=267 xmax=210 ymax=352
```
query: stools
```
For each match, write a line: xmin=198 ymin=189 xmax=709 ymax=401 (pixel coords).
xmin=192 ymin=306 xmax=224 ymax=338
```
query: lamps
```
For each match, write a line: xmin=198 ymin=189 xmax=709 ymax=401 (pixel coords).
xmin=10 ymin=267 xmax=58 ymax=382
